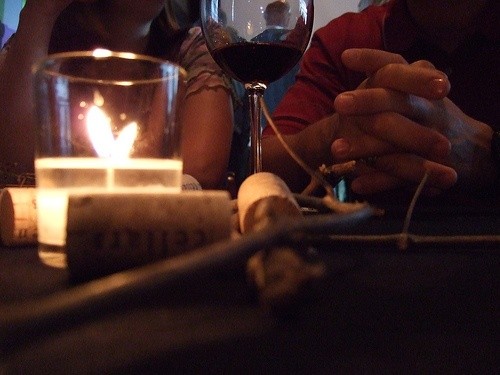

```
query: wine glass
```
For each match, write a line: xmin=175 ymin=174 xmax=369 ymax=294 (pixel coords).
xmin=200 ymin=0 xmax=314 ymax=177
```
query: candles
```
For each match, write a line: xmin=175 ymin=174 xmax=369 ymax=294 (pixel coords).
xmin=35 ymin=102 xmax=185 ymax=272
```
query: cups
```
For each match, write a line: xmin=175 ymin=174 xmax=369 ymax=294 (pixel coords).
xmin=33 ymin=49 xmax=180 ymax=272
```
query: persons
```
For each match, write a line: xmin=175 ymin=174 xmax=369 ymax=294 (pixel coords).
xmin=238 ymin=0 xmax=305 ymax=129
xmin=0 ymin=0 xmax=235 ymax=192
xmin=260 ymin=0 xmax=499 ymax=215
